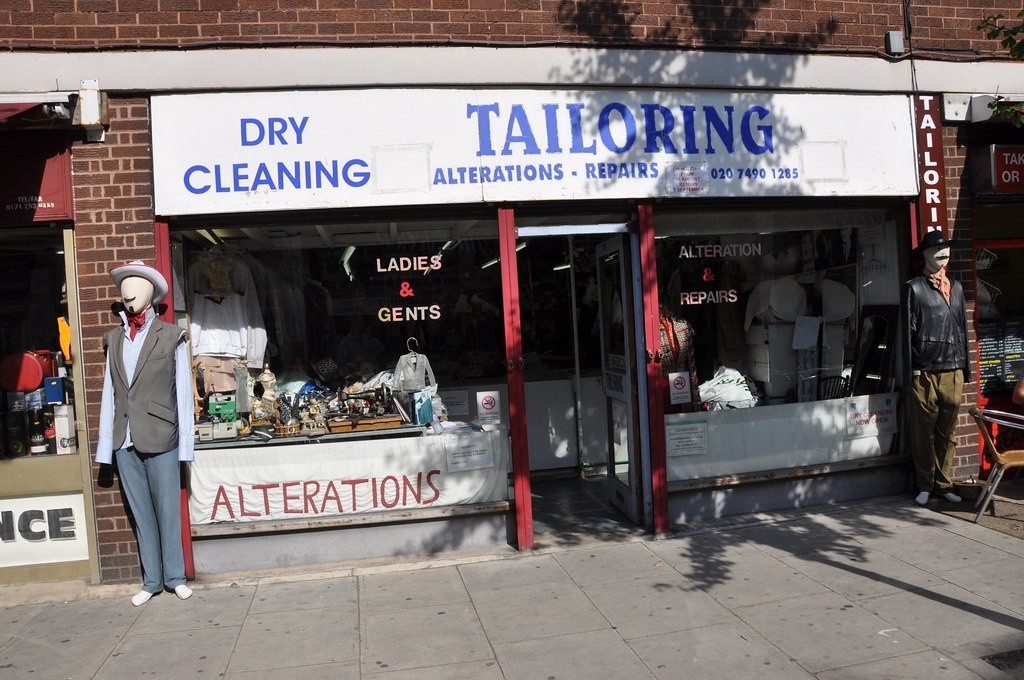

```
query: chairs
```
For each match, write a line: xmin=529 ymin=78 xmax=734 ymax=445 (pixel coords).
xmin=967 ymin=407 xmax=1024 ymax=523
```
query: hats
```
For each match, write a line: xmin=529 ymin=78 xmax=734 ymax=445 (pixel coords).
xmin=915 ymin=229 xmax=955 ymax=254
xmin=108 ymin=260 xmax=169 ymax=306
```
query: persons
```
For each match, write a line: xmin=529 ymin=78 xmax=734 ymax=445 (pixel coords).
xmin=260 ymin=363 xmax=277 ymax=403
xmin=903 ymin=229 xmax=971 ymax=505
xmin=95 ymin=259 xmax=195 ymax=608
xmin=233 ymin=358 xmax=251 ymax=413
